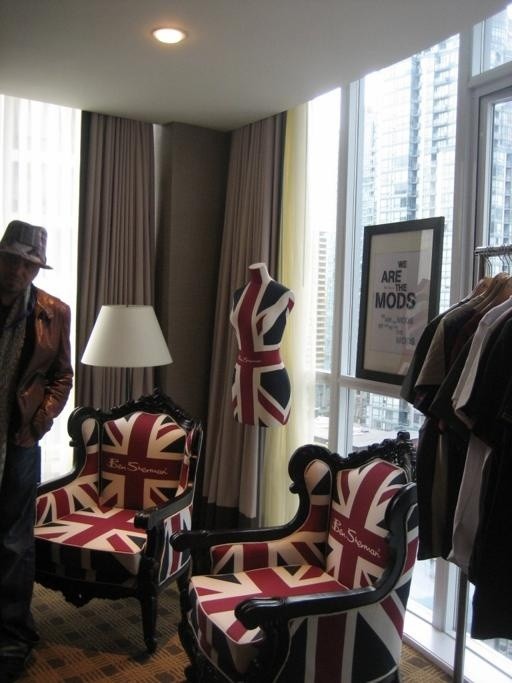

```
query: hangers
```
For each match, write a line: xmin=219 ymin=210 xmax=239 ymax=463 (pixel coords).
xmin=459 ymin=246 xmax=512 ymax=319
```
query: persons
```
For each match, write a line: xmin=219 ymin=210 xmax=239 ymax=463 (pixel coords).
xmin=0 ymin=221 xmax=73 ymax=683
xmin=229 ymin=263 xmax=295 ymax=428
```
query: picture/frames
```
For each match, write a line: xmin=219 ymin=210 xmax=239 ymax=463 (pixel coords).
xmin=356 ymin=217 xmax=444 ymax=381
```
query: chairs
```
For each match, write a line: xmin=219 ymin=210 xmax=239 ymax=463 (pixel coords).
xmin=34 ymin=391 xmax=203 ymax=654
xmin=170 ymin=430 xmax=422 ymax=681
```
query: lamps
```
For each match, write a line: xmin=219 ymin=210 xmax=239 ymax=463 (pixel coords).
xmin=80 ymin=304 xmax=174 ymax=402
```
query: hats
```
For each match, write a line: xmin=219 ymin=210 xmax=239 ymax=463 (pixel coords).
xmin=0 ymin=221 xmax=51 ymax=270
